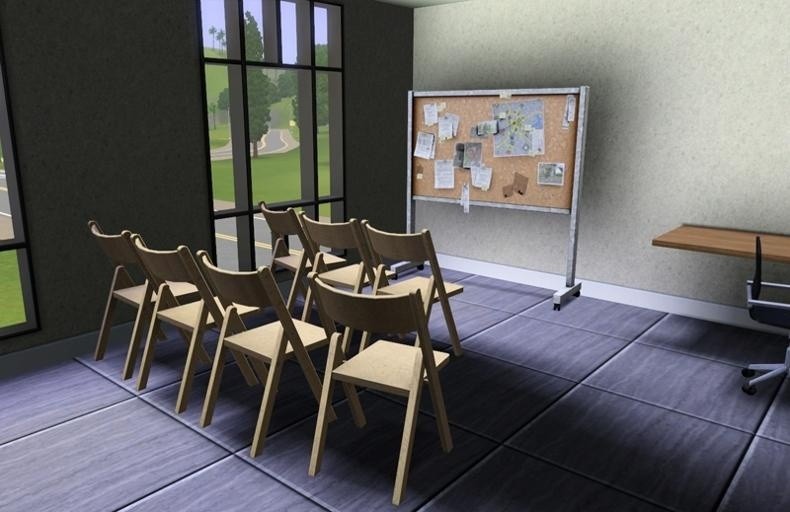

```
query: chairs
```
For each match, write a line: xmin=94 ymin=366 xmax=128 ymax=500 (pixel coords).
xmin=85 ymin=199 xmax=466 ymax=508
xmin=740 ymin=233 xmax=790 ymax=397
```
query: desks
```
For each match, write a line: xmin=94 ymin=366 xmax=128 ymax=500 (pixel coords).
xmin=646 ymin=222 xmax=790 ymax=264
